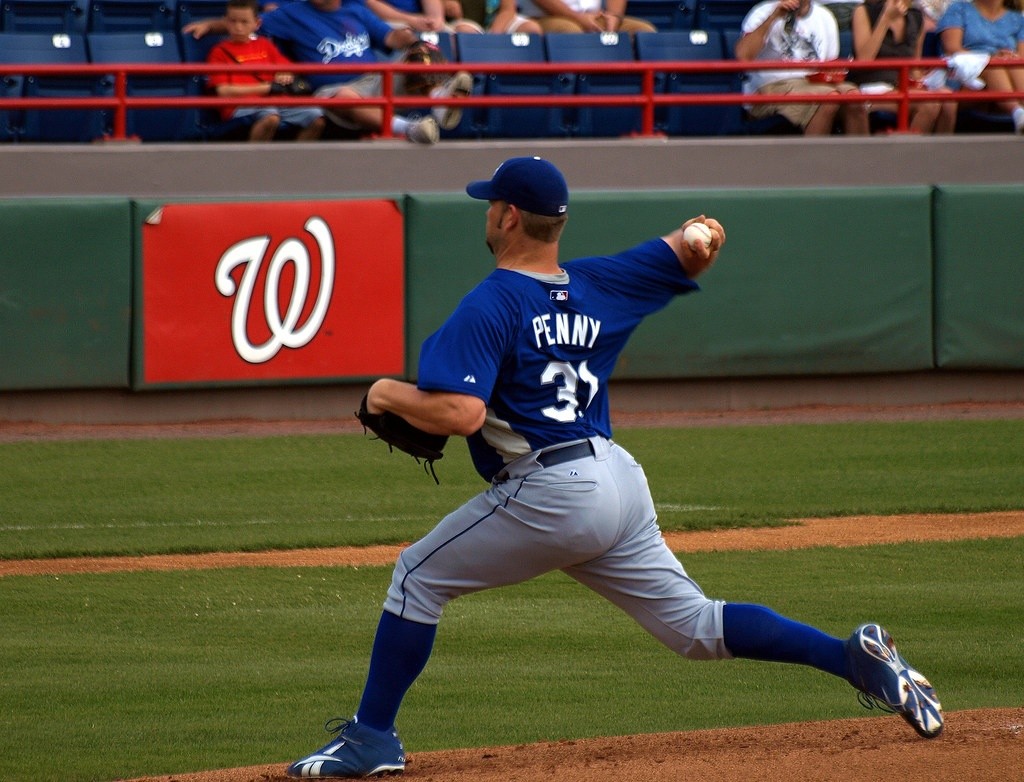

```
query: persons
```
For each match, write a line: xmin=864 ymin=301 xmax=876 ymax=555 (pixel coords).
xmin=935 ymin=0 xmax=1024 ymax=134
xmin=521 ymin=0 xmax=660 ymax=32
xmin=446 ymin=0 xmax=543 ymax=34
xmin=366 ymin=0 xmax=457 ymax=60
xmin=736 ymin=0 xmax=870 ymax=136
xmin=913 ymin=0 xmax=953 ymax=55
xmin=852 ymin=0 xmax=958 ymax=136
xmin=182 ymin=0 xmax=473 ymax=142
xmin=285 ymin=156 xmax=946 ymax=777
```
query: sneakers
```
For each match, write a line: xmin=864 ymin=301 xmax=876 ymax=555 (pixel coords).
xmin=286 ymin=715 xmax=405 ymax=778
xmin=846 ymin=624 xmax=944 ymax=739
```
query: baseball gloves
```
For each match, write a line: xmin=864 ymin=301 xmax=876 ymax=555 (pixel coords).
xmin=356 ymin=389 xmax=450 ymax=465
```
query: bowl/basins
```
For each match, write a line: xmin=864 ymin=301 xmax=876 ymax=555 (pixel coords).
xmin=805 ymin=70 xmax=848 ymax=84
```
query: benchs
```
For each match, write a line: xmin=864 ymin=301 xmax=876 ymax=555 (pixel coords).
xmin=1 ymin=31 xmax=1014 ymax=141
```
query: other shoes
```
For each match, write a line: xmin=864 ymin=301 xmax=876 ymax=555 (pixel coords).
xmin=405 ymin=119 xmax=439 ymax=144
xmin=432 ymin=73 xmax=472 ymax=132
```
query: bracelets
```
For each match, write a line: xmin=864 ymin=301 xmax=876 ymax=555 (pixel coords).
xmin=613 ymin=13 xmax=623 ymax=32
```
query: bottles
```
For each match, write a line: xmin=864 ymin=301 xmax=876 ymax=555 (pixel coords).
xmin=783 ymin=10 xmax=796 ymax=35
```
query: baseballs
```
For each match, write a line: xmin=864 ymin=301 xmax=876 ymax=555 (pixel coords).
xmin=683 ymin=223 xmax=712 ymax=252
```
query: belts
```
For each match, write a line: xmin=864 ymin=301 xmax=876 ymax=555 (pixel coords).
xmin=501 ymin=437 xmax=614 ymax=482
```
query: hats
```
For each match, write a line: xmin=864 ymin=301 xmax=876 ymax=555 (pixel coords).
xmin=466 ymin=157 xmax=569 ymax=217
